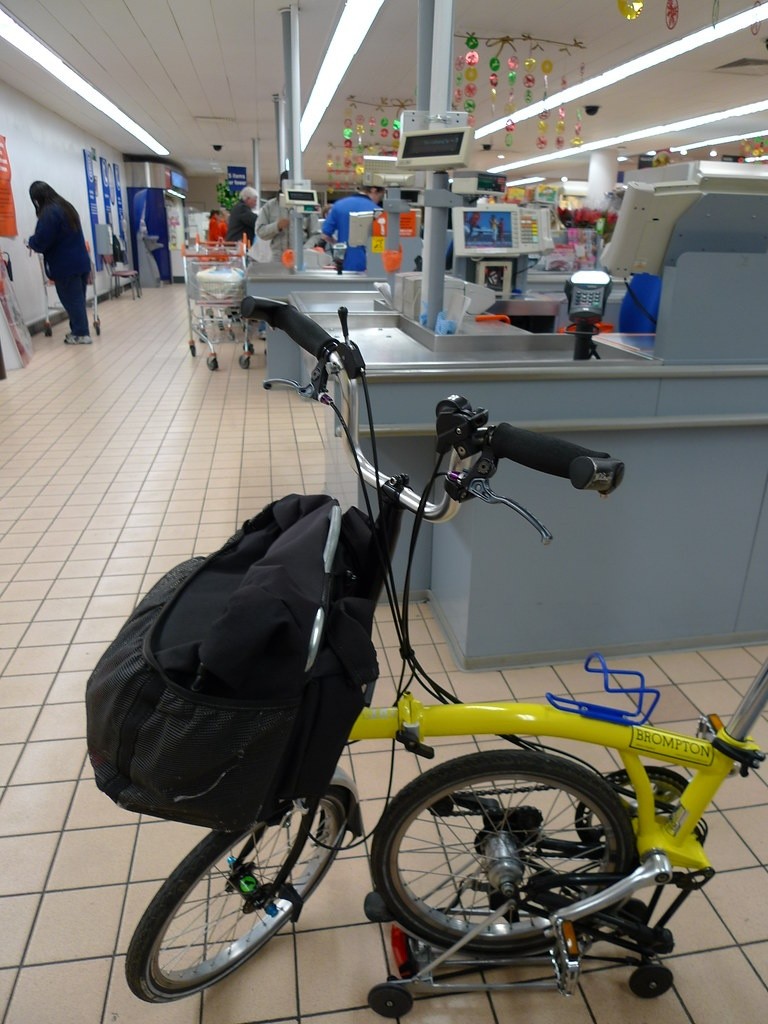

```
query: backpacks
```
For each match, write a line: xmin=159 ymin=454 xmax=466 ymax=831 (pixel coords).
xmin=85 ymin=491 xmax=405 ymax=827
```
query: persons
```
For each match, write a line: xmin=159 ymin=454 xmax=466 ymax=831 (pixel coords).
xmin=24 ymin=181 xmax=91 ymax=344
xmin=206 ymin=211 xmax=227 ymax=258
xmin=322 ymin=204 xmax=338 ymax=249
xmin=465 ymin=212 xmax=504 ymax=246
xmin=247 ymin=239 xmax=271 ymax=339
xmin=227 ymin=187 xmax=258 ymax=248
xmin=321 ymin=185 xmax=385 ymax=274
xmin=255 ymin=170 xmax=322 ymax=264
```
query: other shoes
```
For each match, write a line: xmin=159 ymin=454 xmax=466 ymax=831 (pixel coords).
xmin=64 ymin=333 xmax=91 ymax=344
xmin=259 ymin=330 xmax=266 ymax=341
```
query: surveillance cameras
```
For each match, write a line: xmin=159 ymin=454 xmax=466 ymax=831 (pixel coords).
xmin=585 ymin=105 xmax=598 ymax=116
xmin=213 ymin=145 xmax=222 ymax=151
xmin=483 ymin=144 xmax=492 ymax=150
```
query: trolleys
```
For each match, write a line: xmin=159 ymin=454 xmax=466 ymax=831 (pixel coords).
xmin=179 ymin=229 xmax=257 ymax=373
xmin=34 ymin=240 xmax=103 ymax=338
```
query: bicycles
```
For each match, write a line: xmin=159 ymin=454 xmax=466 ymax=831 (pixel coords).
xmin=124 ymin=296 xmax=767 ymax=1020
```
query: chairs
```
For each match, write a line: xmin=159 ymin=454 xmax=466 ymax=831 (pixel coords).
xmin=103 ymin=256 xmax=142 ymax=300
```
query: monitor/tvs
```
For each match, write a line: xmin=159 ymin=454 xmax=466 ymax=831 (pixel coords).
xmin=463 ymin=211 xmax=513 ymax=249
xmin=170 ymin=171 xmax=189 ymax=193
xmin=348 ymin=209 xmax=421 ymax=247
xmin=606 ymin=170 xmax=768 ymax=277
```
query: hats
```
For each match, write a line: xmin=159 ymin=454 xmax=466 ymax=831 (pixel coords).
xmin=362 ymin=174 xmax=386 ymax=186
